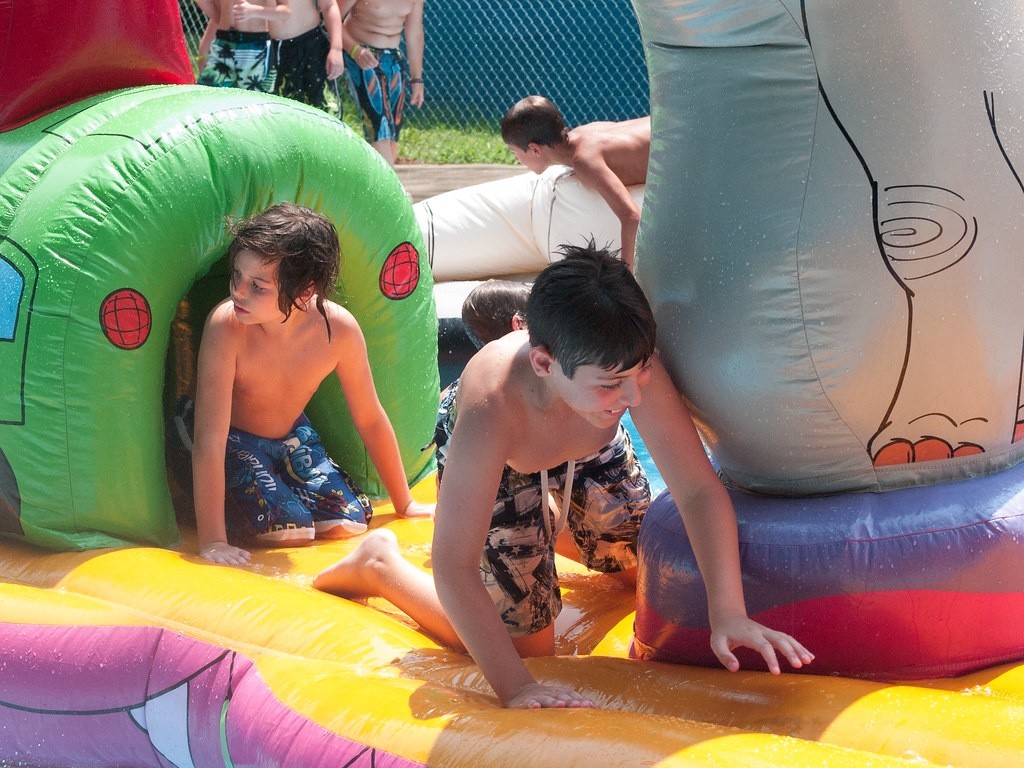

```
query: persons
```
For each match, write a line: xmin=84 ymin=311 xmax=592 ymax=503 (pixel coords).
xmin=462 ymin=277 xmax=533 ymax=355
xmin=501 ymin=96 xmax=651 ymax=271
xmin=192 ymin=0 xmax=427 ymax=167
xmin=167 ymin=199 xmax=433 ymax=569
xmin=310 ymin=242 xmax=817 ymax=710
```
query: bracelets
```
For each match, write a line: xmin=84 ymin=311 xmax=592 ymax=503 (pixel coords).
xmin=350 ymin=44 xmax=361 ymax=58
xmin=196 ymin=54 xmax=208 ymax=62
xmin=411 ymin=78 xmax=424 ymax=84
